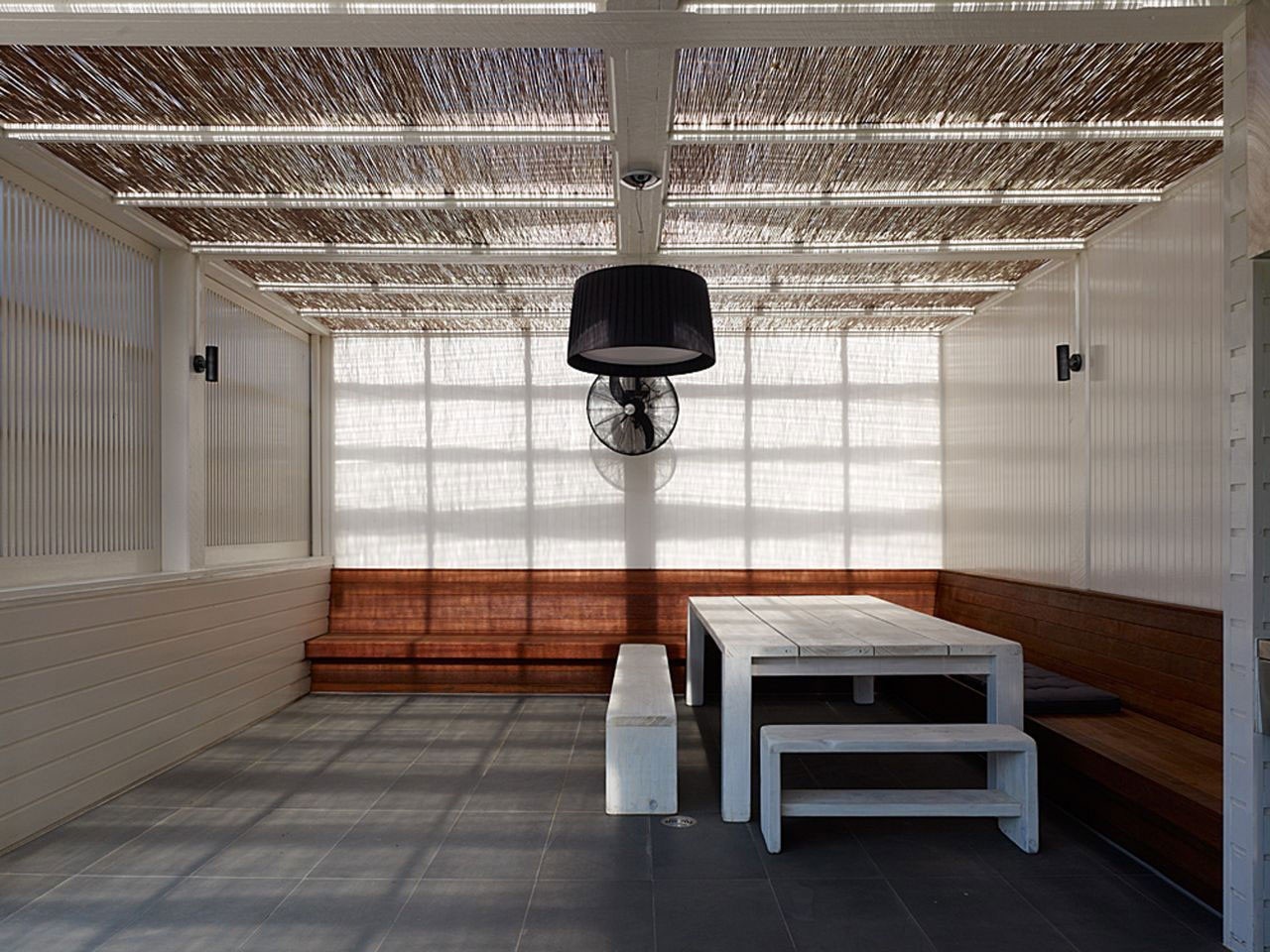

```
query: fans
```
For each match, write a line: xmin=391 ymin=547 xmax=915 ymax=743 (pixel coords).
xmin=586 ymin=375 xmax=679 ymax=456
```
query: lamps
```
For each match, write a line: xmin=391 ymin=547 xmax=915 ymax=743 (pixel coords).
xmin=1056 ymin=345 xmax=1082 ymax=380
xmin=193 ymin=346 xmax=218 ymax=382
xmin=567 ymin=171 xmax=716 ymax=377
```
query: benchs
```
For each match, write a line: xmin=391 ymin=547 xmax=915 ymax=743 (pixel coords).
xmin=606 ymin=643 xmax=677 ymax=814
xmin=304 ymin=569 xmax=939 ymax=698
xmin=760 ymin=724 xmax=1039 ymax=854
xmin=934 ymin=568 xmax=1236 ymax=919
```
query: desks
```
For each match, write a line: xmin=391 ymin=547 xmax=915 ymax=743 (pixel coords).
xmin=686 ymin=596 xmax=1024 ymax=822
